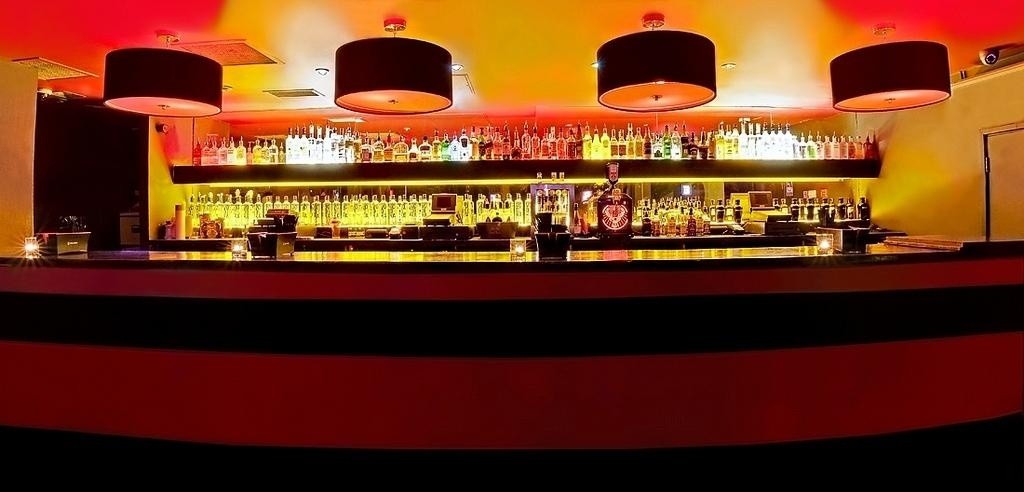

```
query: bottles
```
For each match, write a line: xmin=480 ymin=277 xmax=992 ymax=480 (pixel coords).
xmin=570 ymin=201 xmax=582 ymax=236
xmin=188 ymin=190 xmax=532 ymax=226
xmin=586 ymin=183 xmax=598 ymax=232
xmin=774 ymin=197 xmax=872 ymax=221
xmin=193 ymin=121 xmax=880 ymax=166
xmin=174 ymin=204 xmax=188 ymax=241
xmin=165 ymin=220 xmax=173 ymax=240
xmin=330 ymin=217 xmax=339 ymax=237
xmin=637 ymin=196 xmax=744 ymax=236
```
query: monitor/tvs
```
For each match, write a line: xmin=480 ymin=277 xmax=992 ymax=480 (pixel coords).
xmin=431 ymin=194 xmax=456 ymax=214
xmin=748 ymin=191 xmax=775 ymax=210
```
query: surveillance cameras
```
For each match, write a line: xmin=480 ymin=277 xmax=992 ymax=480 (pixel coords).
xmin=978 ymin=49 xmax=1000 ymax=67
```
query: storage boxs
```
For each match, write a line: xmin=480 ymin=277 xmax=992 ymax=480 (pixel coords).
xmin=245 ymin=231 xmax=299 ymax=260
xmin=813 ymin=225 xmax=871 ymax=255
xmin=35 ymin=231 xmax=92 ymax=256
xmin=533 ymin=229 xmax=574 ymax=261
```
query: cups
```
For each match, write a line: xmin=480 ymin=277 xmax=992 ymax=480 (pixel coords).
xmin=535 ymin=211 xmax=551 ymax=232
xmin=230 ymin=239 xmax=249 ymax=261
xmin=510 ymin=239 xmax=528 ymax=262
xmin=550 ymin=171 xmax=564 ymax=183
xmin=24 ymin=237 xmax=39 ymax=258
xmin=536 ymin=171 xmax=542 ymax=183
xmin=815 ymin=233 xmax=834 ymax=255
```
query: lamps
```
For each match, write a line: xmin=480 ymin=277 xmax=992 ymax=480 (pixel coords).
xmin=828 ymin=22 xmax=951 ymax=114
xmin=596 ymin=13 xmax=719 ymax=112
xmin=333 ymin=18 xmax=454 ymax=116
xmin=101 ymin=32 xmax=224 ymax=119
